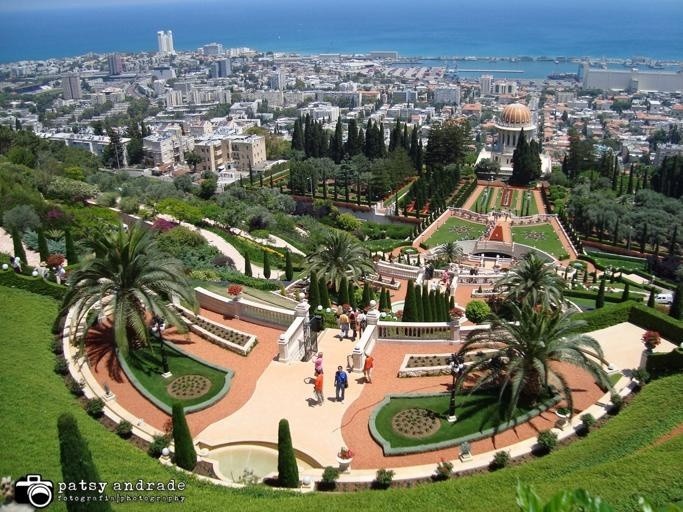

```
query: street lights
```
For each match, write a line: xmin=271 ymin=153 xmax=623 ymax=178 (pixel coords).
xmin=445 ymin=353 xmax=464 ymax=423
xmin=628 ymin=225 xmax=634 ymax=253
xmin=151 ymin=314 xmax=171 ymax=379
xmin=307 ymin=176 xmax=313 ymax=195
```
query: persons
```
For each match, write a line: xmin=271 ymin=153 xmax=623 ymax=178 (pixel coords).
xmin=33 ymin=261 xmax=50 ymax=277
xmin=430 ymin=281 xmax=436 ymax=292
xmin=311 ymin=351 xmax=323 ymax=375
xmin=450 ymin=361 xmax=464 ymax=387
xmin=439 ymin=269 xmax=450 ymax=285
xmin=53 ymin=265 xmax=65 ymax=285
xmin=361 ymin=351 xmax=375 ymax=384
xmin=9 ymin=256 xmax=22 ymax=274
xmin=333 ymin=365 xmax=348 ymax=403
xmin=335 ymin=305 xmax=366 ymax=338
xmin=312 ymin=365 xmax=323 ymax=406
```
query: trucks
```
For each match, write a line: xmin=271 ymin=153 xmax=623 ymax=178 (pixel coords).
xmin=654 ymin=293 xmax=672 ymax=305
